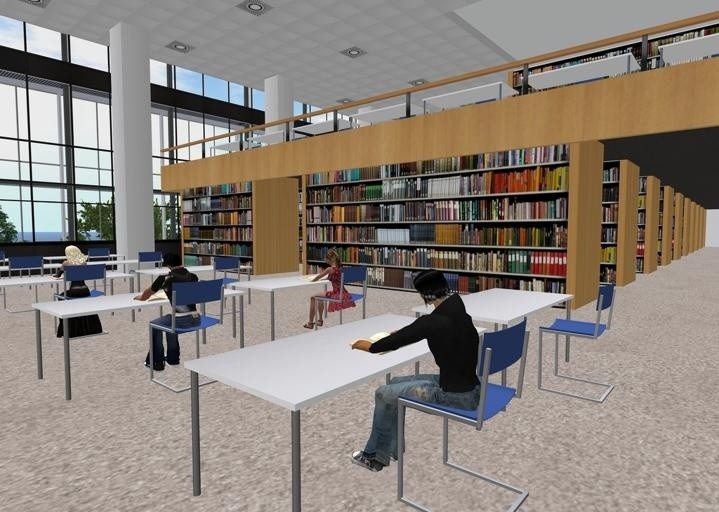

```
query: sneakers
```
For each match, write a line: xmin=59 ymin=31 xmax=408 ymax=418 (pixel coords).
xmin=390 ymin=454 xmax=398 ymax=462
xmin=351 ymin=449 xmax=383 ymax=472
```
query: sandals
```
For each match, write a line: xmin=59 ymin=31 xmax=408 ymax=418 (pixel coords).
xmin=303 ymin=322 xmax=314 ymax=329
xmin=314 ymin=320 xmax=323 ymax=326
